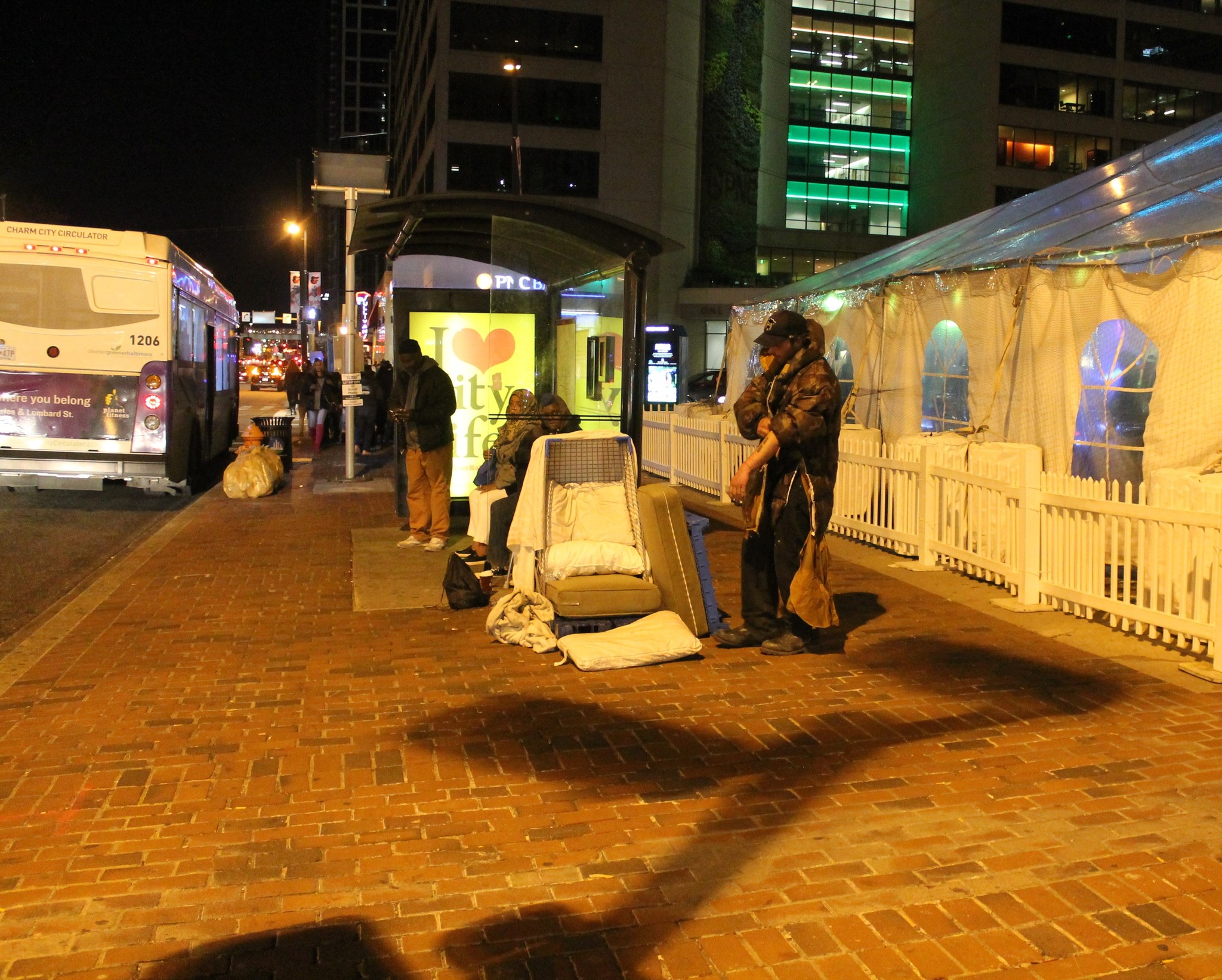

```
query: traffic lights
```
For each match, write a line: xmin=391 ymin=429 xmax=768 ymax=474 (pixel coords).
xmin=298 ymin=344 xmax=302 ymax=348
xmin=262 ymin=338 xmax=267 ymax=342
xmin=282 ymin=343 xmax=286 ymax=347
xmin=306 ymin=308 xmax=321 ymax=320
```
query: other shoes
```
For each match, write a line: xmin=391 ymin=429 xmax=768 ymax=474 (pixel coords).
xmin=475 ymin=566 xmax=512 ymax=579
xmin=460 ymin=551 xmax=487 ymax=566
xmin=714 ymin=619 xmax=777 ymax=647
xmin=760 ymin=625 xmax=825 ymax=656
xmin=454 ymin=545 xmax=474 ymax=557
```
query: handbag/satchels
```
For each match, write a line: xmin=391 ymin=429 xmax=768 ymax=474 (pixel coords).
xmin=785 ymin=530 xmax=840 ymax=628
xmin=741 ymin=463 xmax=767 ymax=530
xmin=444 ymin=552 xmax=487 ymax=610
xmin=473 ymin=446 xmax=498 ymax=487
xmin=324 ymin=394 xmax=335 ymax=405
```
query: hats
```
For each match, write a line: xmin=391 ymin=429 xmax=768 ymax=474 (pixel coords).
xmin=754 ymin=309 xmax=809 ymax=347
xmin=398 ymin=339 xmax=420 ymax=354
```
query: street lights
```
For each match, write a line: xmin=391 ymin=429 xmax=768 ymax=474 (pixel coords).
xmin=282 ymin=219 xmax=308 ymax=374
xmin=503 ymin=60 xmax=523 ymax=196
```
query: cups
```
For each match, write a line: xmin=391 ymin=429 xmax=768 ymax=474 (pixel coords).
xmin=479 ymin=570 xmax=494 ymax=595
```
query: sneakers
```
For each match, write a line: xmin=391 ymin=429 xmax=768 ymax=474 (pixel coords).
xmin=424 ymin=537 xmax=445 ymax=551
xmin=397 ymin=535 xmax=429 ymax=548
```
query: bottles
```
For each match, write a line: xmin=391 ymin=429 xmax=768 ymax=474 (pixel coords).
xmin=545 ymin=570 xmax=571 ymax=582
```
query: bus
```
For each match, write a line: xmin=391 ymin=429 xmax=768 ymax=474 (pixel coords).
xmin=0 ymin=220 xmax=253 ymax=505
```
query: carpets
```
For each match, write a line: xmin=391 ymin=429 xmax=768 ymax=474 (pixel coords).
xmin=350 ymin=526 xmax=485 ymax=611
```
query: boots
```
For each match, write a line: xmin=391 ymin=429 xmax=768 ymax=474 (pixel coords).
xmin=307 ymin=424 xmax=324 ymax=453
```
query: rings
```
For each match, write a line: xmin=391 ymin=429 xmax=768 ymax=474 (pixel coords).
xmin=400 ymin=409 xmax=402 ymax=411
xmin=403 ymin=420 xmax=405 ymax=423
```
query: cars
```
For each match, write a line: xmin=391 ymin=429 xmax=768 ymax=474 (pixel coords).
xmin=238 ymin=354 xmax=304 ymax=391
xmin=687 ymin=368 xmax=726 ymax=403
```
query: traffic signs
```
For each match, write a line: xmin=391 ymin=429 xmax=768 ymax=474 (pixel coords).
xmin=241 ymin=312 xmax=250 ymax=322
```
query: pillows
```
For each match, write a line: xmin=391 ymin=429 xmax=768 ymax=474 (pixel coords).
xmin=557 ymin=610 xmax=703 ymax=672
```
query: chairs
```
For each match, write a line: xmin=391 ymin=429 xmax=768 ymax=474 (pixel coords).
xmin=504 ymin=429 xmax=663 ymax=635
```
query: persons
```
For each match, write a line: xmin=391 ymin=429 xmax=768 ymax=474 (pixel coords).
xmin=364 ymin=356 xmax=393 ymax=448
xmin=455 ymin=389 xmax=542 ymax=566
xmin=282 ymin=359 xmax=341 ymax=456
xmin=486 ymin=393 xmax=583 ymax=578
xmin=353 ymin=371 xmax=383 ymax=455
xmin=386 ymin=339 xmax=457 ymax=552
xmin=713 ymin=310 xmax=843 ymax=656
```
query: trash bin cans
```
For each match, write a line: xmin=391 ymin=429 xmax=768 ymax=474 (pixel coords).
xmin=249 ymin=417 xmax=295 ymax=473
